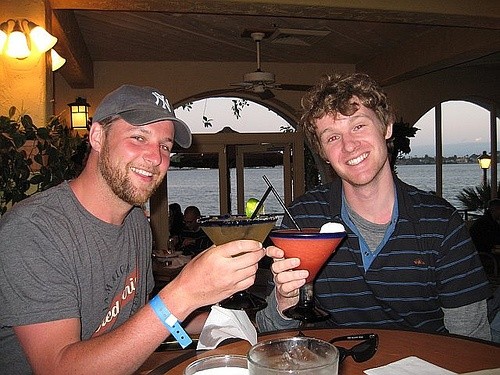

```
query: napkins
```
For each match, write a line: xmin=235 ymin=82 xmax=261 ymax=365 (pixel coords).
xmin=363 ymin=356 xmax=457 ymax=375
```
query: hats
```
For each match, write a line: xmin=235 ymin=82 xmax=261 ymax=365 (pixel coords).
xmin=93 ymin=84 xmax=192 ymax=149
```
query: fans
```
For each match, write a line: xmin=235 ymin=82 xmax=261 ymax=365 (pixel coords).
xmin=220 ymin=33 xmax=283 ymax=93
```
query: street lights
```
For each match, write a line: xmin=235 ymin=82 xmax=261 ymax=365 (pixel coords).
xmin=478 ymin=150 xmax=492 ymax=186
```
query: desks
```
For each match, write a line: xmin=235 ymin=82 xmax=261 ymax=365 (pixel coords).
xmin=131 ymin=327 xmax=500 ymax=375
xmin=152 ymin=259 xmax=187 ymax=283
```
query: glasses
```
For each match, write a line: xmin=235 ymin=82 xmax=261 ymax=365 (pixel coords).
xmin=297 ymin=332 xmax=378 ymax=362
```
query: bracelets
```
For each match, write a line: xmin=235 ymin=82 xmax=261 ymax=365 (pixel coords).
xmin=150 ymin=295 xmax=192 ymax=348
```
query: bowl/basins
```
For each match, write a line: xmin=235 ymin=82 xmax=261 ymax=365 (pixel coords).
xmin=157 ymin=254 xmax=178 ymax=263
xmin=183 ymin=354 xmax=249 ymax=375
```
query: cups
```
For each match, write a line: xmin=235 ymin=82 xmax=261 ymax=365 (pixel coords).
xmin=247 ymin=336 xmax=340 ymax=375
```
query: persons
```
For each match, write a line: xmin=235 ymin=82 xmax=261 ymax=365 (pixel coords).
xmin=168 ymin=203 xmax=212 ymax=255
xmin=256 ymin=73 xmax=492 ymax=341
xmin=0 ymin=84 xmax=267 ymax=375
xmin=469 ymin=198 xmax=500 ymax=277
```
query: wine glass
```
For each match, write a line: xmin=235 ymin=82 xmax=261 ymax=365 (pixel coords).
xmin=196 ymin=213 xmax=278 ymax=310
xmin=269 ymin=227 xmax=347 ymax=322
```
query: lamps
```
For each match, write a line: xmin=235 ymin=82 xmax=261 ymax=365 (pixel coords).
xmin=477 ymin=151 xmax=492 ymax=169
xmin=67 ymin=97 xmax=91 ymax=130
xmin=0 ymin=19 xmax=67 ymax=72
